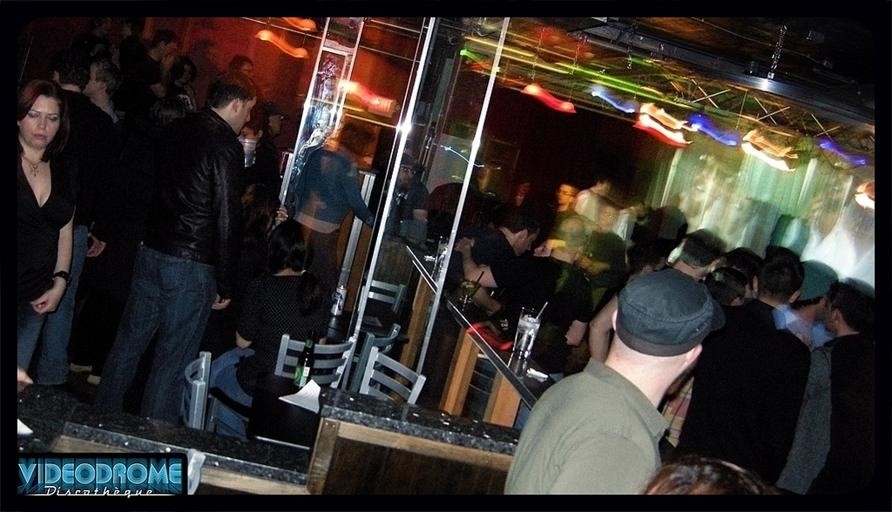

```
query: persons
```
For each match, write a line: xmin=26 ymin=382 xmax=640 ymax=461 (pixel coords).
xmin=19 ymin=20 xmax=877 ymax=498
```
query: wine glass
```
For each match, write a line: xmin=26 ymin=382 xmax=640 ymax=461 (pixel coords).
xmin=459 ymin=279 xmax=481 ymax=302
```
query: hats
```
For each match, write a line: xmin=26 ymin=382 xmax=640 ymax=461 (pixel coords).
xmin=616 ymin=268 xmax=725 ymax=356
xmin=262 ymin=100 xmax=287 ymax=119
xmin=798 ymin=260 xmax=838 ymax=301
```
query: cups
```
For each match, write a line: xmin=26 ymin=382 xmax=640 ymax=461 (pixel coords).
xmin=513 ymin=305 xmax=541 ymax=362
xmin=236 ymin=137 xmax=257 ymax=168
xmin=432 ymin=235 xmax=449 ymax=272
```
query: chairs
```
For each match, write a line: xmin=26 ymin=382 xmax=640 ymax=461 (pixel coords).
xmin=359 ymin=349 xmax=428 ymax=413
xmin=179 ymin=349 xmax=212 ymax=432
xmin=350 ymin=322 xmax=403 ymax=392
xmin=274 ymin=328 xmax=357 ymax=390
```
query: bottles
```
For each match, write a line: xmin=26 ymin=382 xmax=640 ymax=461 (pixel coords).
xmin=293 ymin=328 xmax=319 ymax=389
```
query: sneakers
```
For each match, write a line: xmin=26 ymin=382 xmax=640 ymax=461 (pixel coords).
xmin=71 ymin=356 xmax=93 ymax=372
xmin=88 ymin=368 xmax=105 ymax=384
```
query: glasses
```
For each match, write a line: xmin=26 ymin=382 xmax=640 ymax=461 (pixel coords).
xmin=399 ymin=166 xmax=417 ymax=176
xmin=558 ymin=189 xmax=575 ymax=196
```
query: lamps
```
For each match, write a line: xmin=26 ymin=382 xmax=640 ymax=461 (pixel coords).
xmin=283 ymin=17 xmax=319 ymax=33
xmin=639 ymin=102 xmax=690 ymax=131
xmin=687 ymin=112 xmax=740 ymax=148
xmin=520 ymin=82 xmax=576 ymax=114
xmin=852 ymin=178 xmax=876 ymax=212
xmin=814 ymin=136 xmax=871 ymax=170
xmin=632 ymin=114 xmax=695 ymax=148
xmin=253 ymin=29 xmax=309 ymax=60
xmin=740 ymin=129 xmax=800 ymax=171
xmin=588 ymin=83 xmax=638 ymax=114
xmin=335 ymin=77 xmax=398 ymax=117
